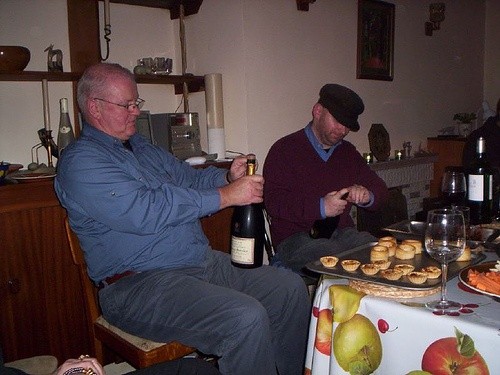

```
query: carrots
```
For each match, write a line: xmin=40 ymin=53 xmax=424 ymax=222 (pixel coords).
xmin=467 ymin=269 xmax=500 ymax=296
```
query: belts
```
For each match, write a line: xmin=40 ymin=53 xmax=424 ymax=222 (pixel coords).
xmin=98 ymin=270 xmax=131 ymax=290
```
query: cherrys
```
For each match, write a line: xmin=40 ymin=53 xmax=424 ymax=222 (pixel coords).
xmin=433 ymin=303 xmax=491 ymax=318
xmin=378 ymin=319 xmax=398 ymax=333
xmin=313 ymin=307 xmax=319 ymax=317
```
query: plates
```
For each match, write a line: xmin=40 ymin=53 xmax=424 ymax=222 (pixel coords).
xmin=459 ymin=262 xmax=500 ymax=298
xmin=9 ymin=171 xmax=56 ymax=183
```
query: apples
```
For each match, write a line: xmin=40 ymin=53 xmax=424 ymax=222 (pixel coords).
xmin=314 ymin=284 xmax=383 ymax=375
xmin=407 ymin=337 xmax=489 ymax=375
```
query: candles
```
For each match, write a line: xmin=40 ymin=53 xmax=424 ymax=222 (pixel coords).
xmin=362 ymin=152 xmax=374 ymax=164
xmin=395 ymin=150 xmax=404 ymax=160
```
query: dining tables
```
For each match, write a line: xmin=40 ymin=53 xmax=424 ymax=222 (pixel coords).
xmin=305 ymin=221 xmax=500 ymax=375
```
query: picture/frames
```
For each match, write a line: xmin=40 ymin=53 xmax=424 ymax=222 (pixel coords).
xmin=356 ymin=0 xmax=396 ymax=81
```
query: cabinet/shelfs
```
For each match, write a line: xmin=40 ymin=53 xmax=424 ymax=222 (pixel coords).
xmin=0 ymin=0 xmax=232 ymax=369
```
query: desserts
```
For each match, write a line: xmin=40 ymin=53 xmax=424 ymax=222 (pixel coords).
xmin=360 ymin=260 xmax=441 ymax=284
xmin=320 ymin=256 xmax=338 ymax=267
xmin=341 ymin=260 xmax=360 ymax=271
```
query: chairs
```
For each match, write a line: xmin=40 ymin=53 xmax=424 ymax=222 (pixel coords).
xmin=64 ymin=218 xmax=199 ymax=369
xmin=262 ymin=208 xmax=319 ymax=290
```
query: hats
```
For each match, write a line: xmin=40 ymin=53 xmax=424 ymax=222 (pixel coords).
xmin=318 ymin=83 xmax=364 ymax=132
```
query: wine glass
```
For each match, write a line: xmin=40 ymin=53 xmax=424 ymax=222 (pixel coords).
xmin=424 ymin=208 xmax=466 ymax=312
xmin=441 ymin=172 xmax=467 ymax=211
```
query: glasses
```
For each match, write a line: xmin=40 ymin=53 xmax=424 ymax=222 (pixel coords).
xmin=93 ymin=97 xmax=145 ymax=112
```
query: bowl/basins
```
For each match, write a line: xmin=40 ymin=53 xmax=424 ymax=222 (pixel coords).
xmin=0 ymin=45 xmax=30 ymax=72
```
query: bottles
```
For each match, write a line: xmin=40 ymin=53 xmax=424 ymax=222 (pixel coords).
xmin=310 ymin=189 xmax=349 ymax=238
xmin=58 ymin=97 xmax=75 ymax=158
xmin=230 ymin=153 xmax=265 ymax=268
xmin=404 ymin=142 xmax=410 ymax=159
xmin=467 ymin=135 xmax=493 ymax=223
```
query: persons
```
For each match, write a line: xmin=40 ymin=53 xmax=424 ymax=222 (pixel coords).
xmin=51 ymin=354 xmax=106 ymax=375
xmin=54 ymin=62 xmax=312 ymax=375
xmin=261 ymin=84 xmax=390 ymax=286
xmin=462 ymin=99 xmax=500 ymax=212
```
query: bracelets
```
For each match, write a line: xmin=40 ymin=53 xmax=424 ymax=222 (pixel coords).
xmin=226 ymin=172 xmax=231 ymax=183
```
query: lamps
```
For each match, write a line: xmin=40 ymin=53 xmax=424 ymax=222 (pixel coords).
xmin=425 ymin=3 xmax=446 ymax=36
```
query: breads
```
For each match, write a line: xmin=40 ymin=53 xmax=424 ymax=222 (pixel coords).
xmin=371 ymin=236 xmax=422 ymax=262
xmin=433 ymin=240 xmax=471 ymax=261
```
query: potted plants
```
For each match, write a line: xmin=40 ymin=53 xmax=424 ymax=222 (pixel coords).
xmin=453 ymin=112 xmax=478 ymax=136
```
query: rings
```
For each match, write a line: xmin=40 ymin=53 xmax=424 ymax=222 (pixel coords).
xmin=78 ymin=354 xmax=89 ymax=359
xmin=86 ymin=368 xmax=94 ymax=375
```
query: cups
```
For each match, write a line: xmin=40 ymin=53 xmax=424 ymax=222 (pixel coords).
xmin=453 ymin=206 xmax=470 ymax=262
xmin=363 ymin=153 xmax=373 ymax=164
xmin=395 ymin=150 xmax=404 ymax=160
